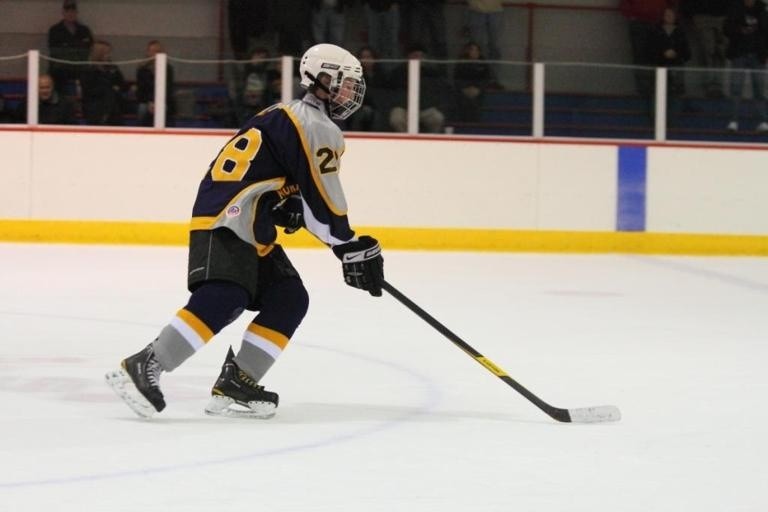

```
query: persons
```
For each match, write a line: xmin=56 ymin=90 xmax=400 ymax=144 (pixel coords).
xmin=618 ymin=2 xmax=768 ymax=131
xmin=228 ymin=1 xmax=505 ymax=133
xmin=0 ymin=1 xmax=177 ymax=127
xmin=120 ymin=43 xmax=384 ymax=411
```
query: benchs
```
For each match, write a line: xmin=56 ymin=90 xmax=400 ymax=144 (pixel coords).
xmin=0 ymin=71 xmax=766 ymax=144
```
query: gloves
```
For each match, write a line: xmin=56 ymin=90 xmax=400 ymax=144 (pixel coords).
xmin=333 ymin=235 xmax=384 ymax=297
xmin=268 ymin=195 xmax=308 ymax=234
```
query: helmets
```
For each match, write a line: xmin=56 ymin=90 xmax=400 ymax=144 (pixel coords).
xmin=298 ymin=42 xmax=368 ymax=122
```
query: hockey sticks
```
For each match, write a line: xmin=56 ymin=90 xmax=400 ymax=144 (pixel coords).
xmin=303 ymin=226 xmax=621 ymax=423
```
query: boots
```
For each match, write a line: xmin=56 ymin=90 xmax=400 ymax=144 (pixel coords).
xmin=211 ymin=344 xmax=280 ymax=409
xmin=120 ymin=336 xmax=167 ymax=412
